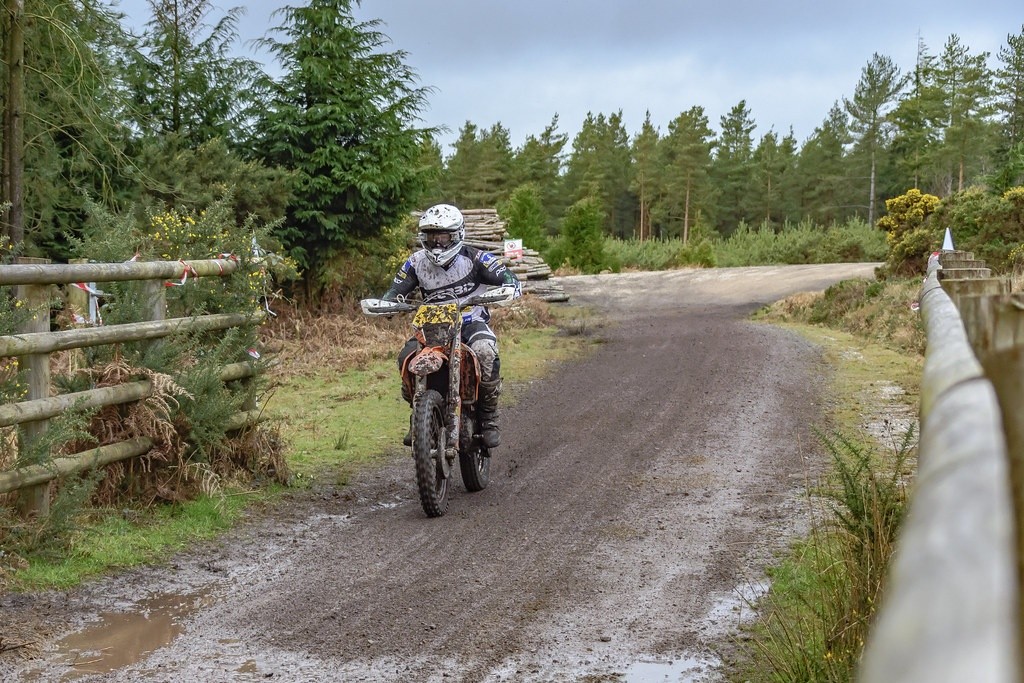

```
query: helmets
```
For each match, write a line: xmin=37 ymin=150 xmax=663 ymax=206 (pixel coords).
xmin=417 ymin=204 xmax=465 ymax=268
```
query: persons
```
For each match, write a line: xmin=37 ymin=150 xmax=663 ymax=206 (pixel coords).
xmin=382 ymin=204 xmax=520 ymax=447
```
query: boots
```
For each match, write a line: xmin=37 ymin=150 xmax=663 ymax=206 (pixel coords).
xmin=478 ymin=377 xmax=502 ymax=449
xmin=403 ymin=402 xmax=414 ymax=447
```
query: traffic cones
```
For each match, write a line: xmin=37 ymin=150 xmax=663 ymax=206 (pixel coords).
xmin=943 ymin=227 xmax=954 ymax=252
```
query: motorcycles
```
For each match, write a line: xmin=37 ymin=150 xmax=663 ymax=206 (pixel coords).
xmin=360 ymin=283 xmax=520 ymax=517
